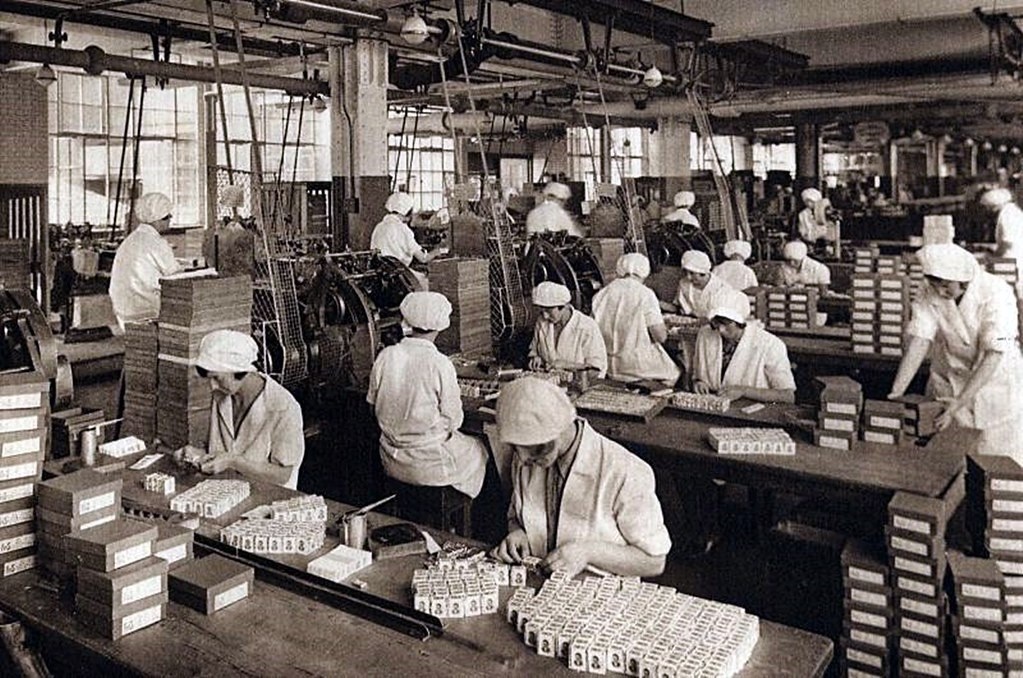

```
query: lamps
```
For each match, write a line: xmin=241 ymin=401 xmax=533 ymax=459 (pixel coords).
xmin=644 ymin=0 xmax=663 ymax=87
xmin=511 ymin=122 xmax=520 ymax=133
xmin=401 ymin=9 xmax=430 ymax=44
xmin=35 ymin=20 xmax=57 ymax=87
xmin=315 ymin=97 xmax=327 ymax=113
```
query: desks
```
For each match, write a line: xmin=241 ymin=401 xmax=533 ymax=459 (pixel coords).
xmin=348 ymin=355 xmax=984 ymax=631
xmin=1 ymin=447 xmax=834 ymax=678
xmin=665 ymin=326 xmax=931 ymax=407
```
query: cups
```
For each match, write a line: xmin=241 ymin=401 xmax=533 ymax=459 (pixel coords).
xmin=342 ymin=510 xmax=367 ymax=549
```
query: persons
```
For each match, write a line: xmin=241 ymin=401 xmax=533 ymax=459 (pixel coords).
xmin=109 ymin=192 xmax=186 ymax=332
xmin=366 ymin=291 xmax=511 ymax=548
xmin=369 ymin=181 xmax=1021 ymax=463
xmin=172 ymin=327 xmax=306 ymax=491
xmin=490 ymin=375 xmax=673 ymax=581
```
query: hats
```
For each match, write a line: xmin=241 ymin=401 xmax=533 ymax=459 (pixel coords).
xmin=680 ymin=250 xmax=711 ymax=274
xmin=196 ymin=329 xmax=259 ymax=373
xmin=616 ymin=252 xmax=651 ymax=278
xmin=542 ymin=181 xmax=572 ymax=199
xmin=706 ymin=288 xmax=751 ymax=325
xmin=915 ymin=243 xmax=978 ymax=282
xmin=133 ymin=192 xmax=170 ymax=222
xmin=979 ymin=189 xmax=1012 ymax=205
xmin=399 ymin=292 xmax=453 ymax=330
xmin=495 ymin=377 xmax=578 ymax=445
xmin=723 ymin=240 xmax=752 ymax=261
xmin=220 ymin=186 xmax=244 ymax=208
xmin=384 ymin=193 xmax=412 ymax=215
xmin=532 ymin=281 xmax=572 ymax=307
xmin=673 ymin=191 xmax=694 ymax=207
xmin=801 ymin=188 xmax=822 ymax=202
xmin=782 ymin=241 xmax=807 ymax=260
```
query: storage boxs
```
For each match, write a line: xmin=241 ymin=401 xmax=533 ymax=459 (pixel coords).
xmin=428 ymin=169 xmax=795 ymax=360
xmin=742 ymin=215 xmax=1023 ymax=678
xmin=0 ymin=240 xmax=254 ymax=641
